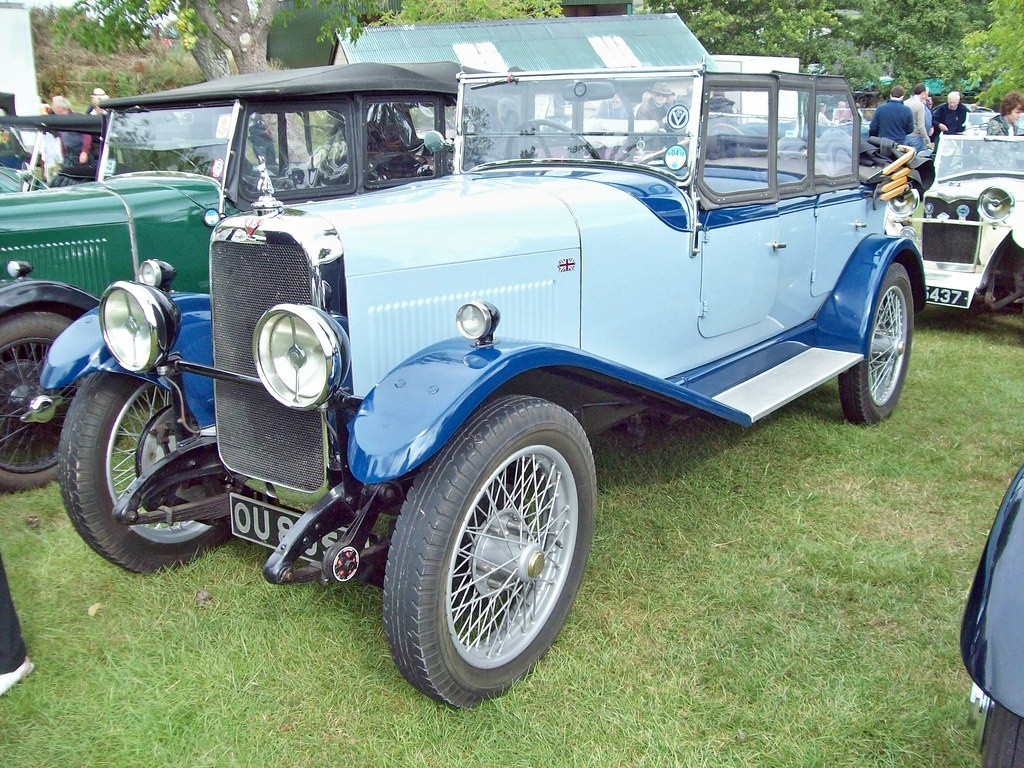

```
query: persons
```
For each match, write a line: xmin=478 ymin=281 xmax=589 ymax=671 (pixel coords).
xmin=903 ymin=85 xmax=930 ymax=153
xmin=0 ymin=88 xmax=110 ymax=183
xmin=0 ymin=552 xmax=34 ymax=698
xmin=612 ymin=81 xmax=693 ymax=150
xmin=921 ymin=87 xmax=934 ymax=143
xmin=710 ymin=92 xmax=736 ymax=159
xmin=869 ymin=85 xmax=914 ymax=145
xmin=986 ymin=91 xmax=1024 ymax=136
xmin=970 ymin=105 xmax=983 ymax=126
xmin=834 ymin=101 xmax=849 ymax=123
xmin=933 ymin=91 xmax=966 ymax=135
xmin=817 ymin=103 xmax=831 ymax=125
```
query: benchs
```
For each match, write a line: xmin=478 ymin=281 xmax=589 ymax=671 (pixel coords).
xmin=702 ymin=168 xmax=798 ymax=193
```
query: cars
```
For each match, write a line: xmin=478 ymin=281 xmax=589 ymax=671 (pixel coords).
xmin=883 ymin=129 xmax=1024 ymax=313
xmin=0 ymin=112 xmax=110 ymax=197
xmin=0 ymin=57 xmax=491 ymax=494
xmin=15 ymin=69 xmax=927 ymax=714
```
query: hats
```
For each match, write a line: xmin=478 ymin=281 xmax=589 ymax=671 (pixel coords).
xmin=90 ymin=87 xmax=110 ymax=99
xmin=651 ymin=82 xmax=672 ymax=96
